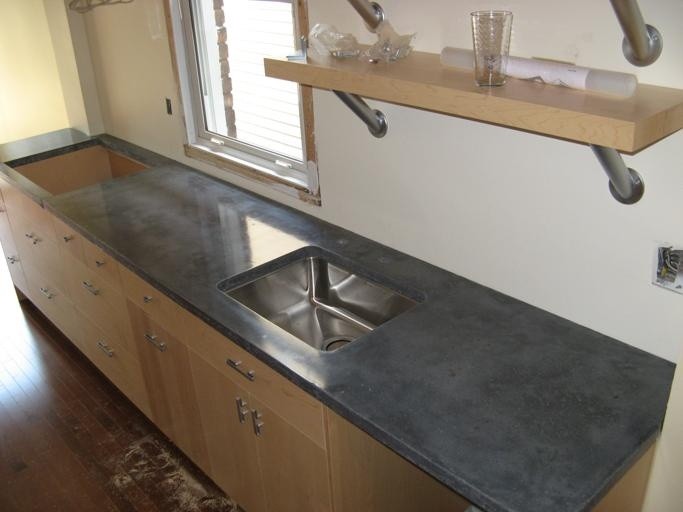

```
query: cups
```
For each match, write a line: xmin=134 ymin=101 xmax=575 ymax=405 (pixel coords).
xmin=470 ymin=10 xmax=513 ymax=88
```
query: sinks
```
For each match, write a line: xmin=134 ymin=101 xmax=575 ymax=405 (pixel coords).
xmin=216 ymin=245 xmax=428 ymax=352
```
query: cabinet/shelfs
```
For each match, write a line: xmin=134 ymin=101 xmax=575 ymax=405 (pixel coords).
xmin=183 ymin=309 xmax=332 ymax=512
xmin=0 ymin=176 xmax=121 ymax=392
xmin=122 ymin=266 xmax=210 ymax=478
